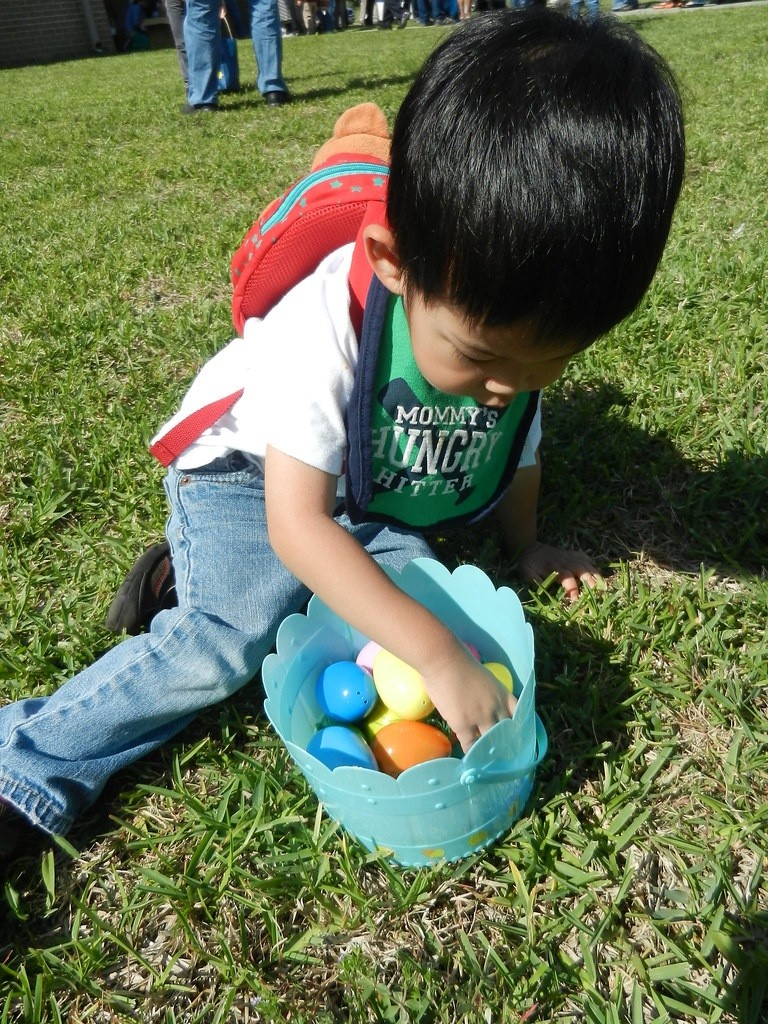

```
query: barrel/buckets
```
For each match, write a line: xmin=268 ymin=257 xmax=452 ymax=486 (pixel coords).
xmin=258 ymin=553 xmax=552 ymax=871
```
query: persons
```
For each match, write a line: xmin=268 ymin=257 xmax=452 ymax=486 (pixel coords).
xmin=0 ymin=0 xmax=693 ymax=956
xmin=276 ymin=0 xmax=715 ymax=39
xmin=180 ymin=0 xmax=292 ymax=117
xmin=163 ymin=0 xmax=227 ymax=97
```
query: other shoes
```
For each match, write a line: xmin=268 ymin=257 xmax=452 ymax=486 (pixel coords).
xmin=178 ymin=104 xmax=217 ymax=114
xmin=264 ymin=91 xmax=287 ymax=107
xmin=612 ymin=0 xmax=706 ymax=12
xmin=281 ymin=10 xmax=491 ymax=38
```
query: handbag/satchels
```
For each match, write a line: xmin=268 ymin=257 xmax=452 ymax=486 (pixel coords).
xmin=216 ymin=15 xmax=240 ymax=93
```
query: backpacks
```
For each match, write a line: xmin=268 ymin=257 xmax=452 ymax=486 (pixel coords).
xmin=229 ymin=103 xmax=542 ymax=530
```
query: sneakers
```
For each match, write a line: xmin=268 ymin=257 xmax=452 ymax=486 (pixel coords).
xmin=108 ymin=543 xmax=181 ymax=636
